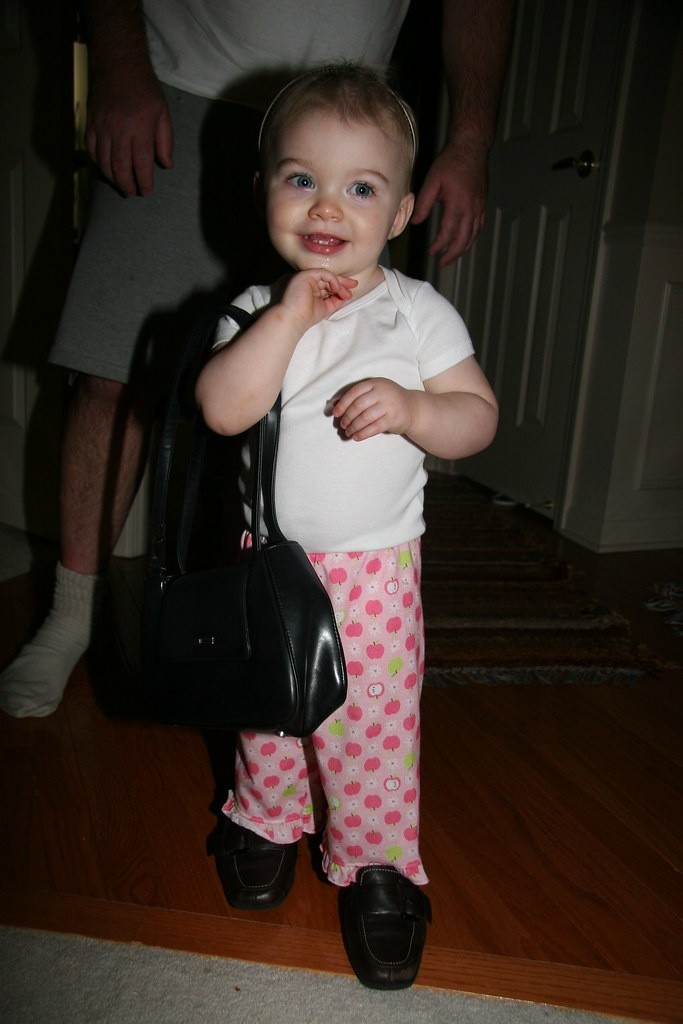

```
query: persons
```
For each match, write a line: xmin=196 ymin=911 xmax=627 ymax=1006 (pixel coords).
xmin=193 ymin=61 xmax=498 ymax=991
xmin=0 ymin=0 xmax=514 ymax=720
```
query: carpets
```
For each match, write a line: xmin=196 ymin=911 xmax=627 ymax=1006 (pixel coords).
xmin=0 ymin=925 xmax=654 ymax=1024
xmin=416 ymin=468 xmax=683 ymax=690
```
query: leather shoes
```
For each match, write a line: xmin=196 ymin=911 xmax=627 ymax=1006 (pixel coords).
xmin=339 ymin=865 xmax=432 ymax=990
xmin=207 ymin=814 xmax=299 ymax=909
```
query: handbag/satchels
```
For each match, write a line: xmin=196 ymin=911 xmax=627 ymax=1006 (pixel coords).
xmin=141 ymin=306 xmax=346 ymax=738
